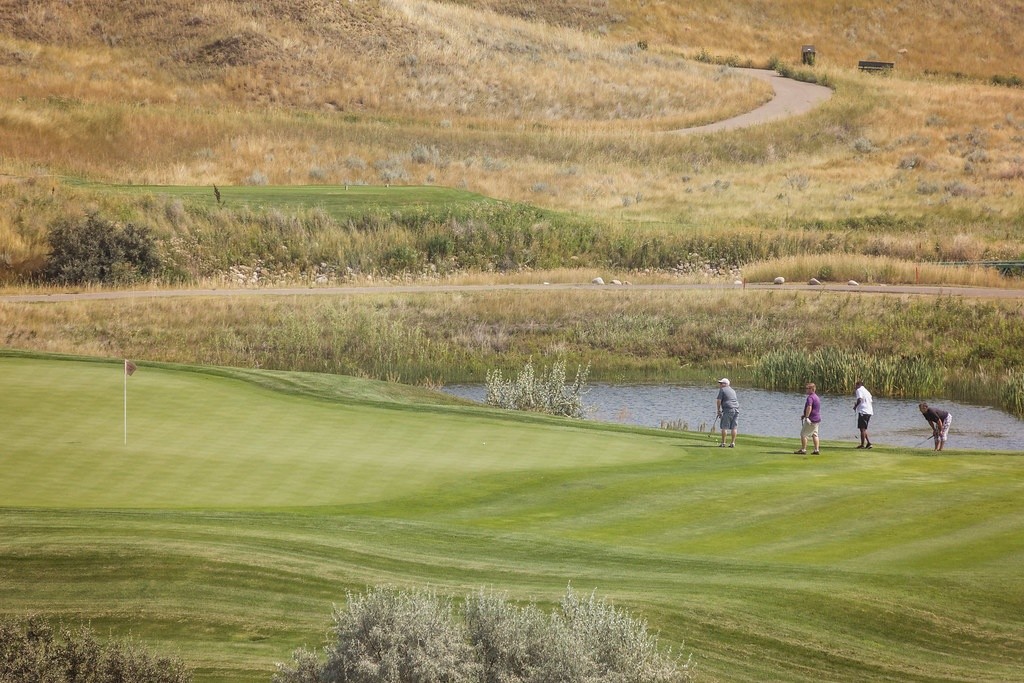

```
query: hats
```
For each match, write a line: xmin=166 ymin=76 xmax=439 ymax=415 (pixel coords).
xmin=718 ymin=378 xmax=730 ymax=385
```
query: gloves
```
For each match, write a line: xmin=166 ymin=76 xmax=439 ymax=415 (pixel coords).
xmin=806 ymin=417 xmax=812 ymax=426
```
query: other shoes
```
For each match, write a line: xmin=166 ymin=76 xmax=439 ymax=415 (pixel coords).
xmin=933 ymin=449 xmax=943 ymax=451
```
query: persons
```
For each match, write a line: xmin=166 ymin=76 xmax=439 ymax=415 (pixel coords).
xmin=716 ymin=378 xmax=739 ymax=447
xmin=794 ymin=383 xmax=822 ymax=455
xmin=919 ymin=402 xmax=952 ymax=451
xmin=852 ymin=381 xmax=873 ymax=449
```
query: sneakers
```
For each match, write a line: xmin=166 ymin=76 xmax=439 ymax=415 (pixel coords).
xmin=856 ymin=442 xmax=872 ymax=449
xmin=794 ymin=449 xmax=807 ymax=454
xmin=728 ymin=443 xmax=735 ymax=448
xmin=811 ymin=451 xmax=820 ymax=455
xmin=719 ymin=443 xmax=726 ymax=447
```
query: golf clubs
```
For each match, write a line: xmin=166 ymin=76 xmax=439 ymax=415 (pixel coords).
xmin=855 ymin=410 xmax=858 ymax=439
xmin=802 ymin=418 xmax=804 ymax=427
xmin=708 ymin=414 xmax=718 ymax=437
xmin=914 ymin=434 xmax=934 ymax=448
